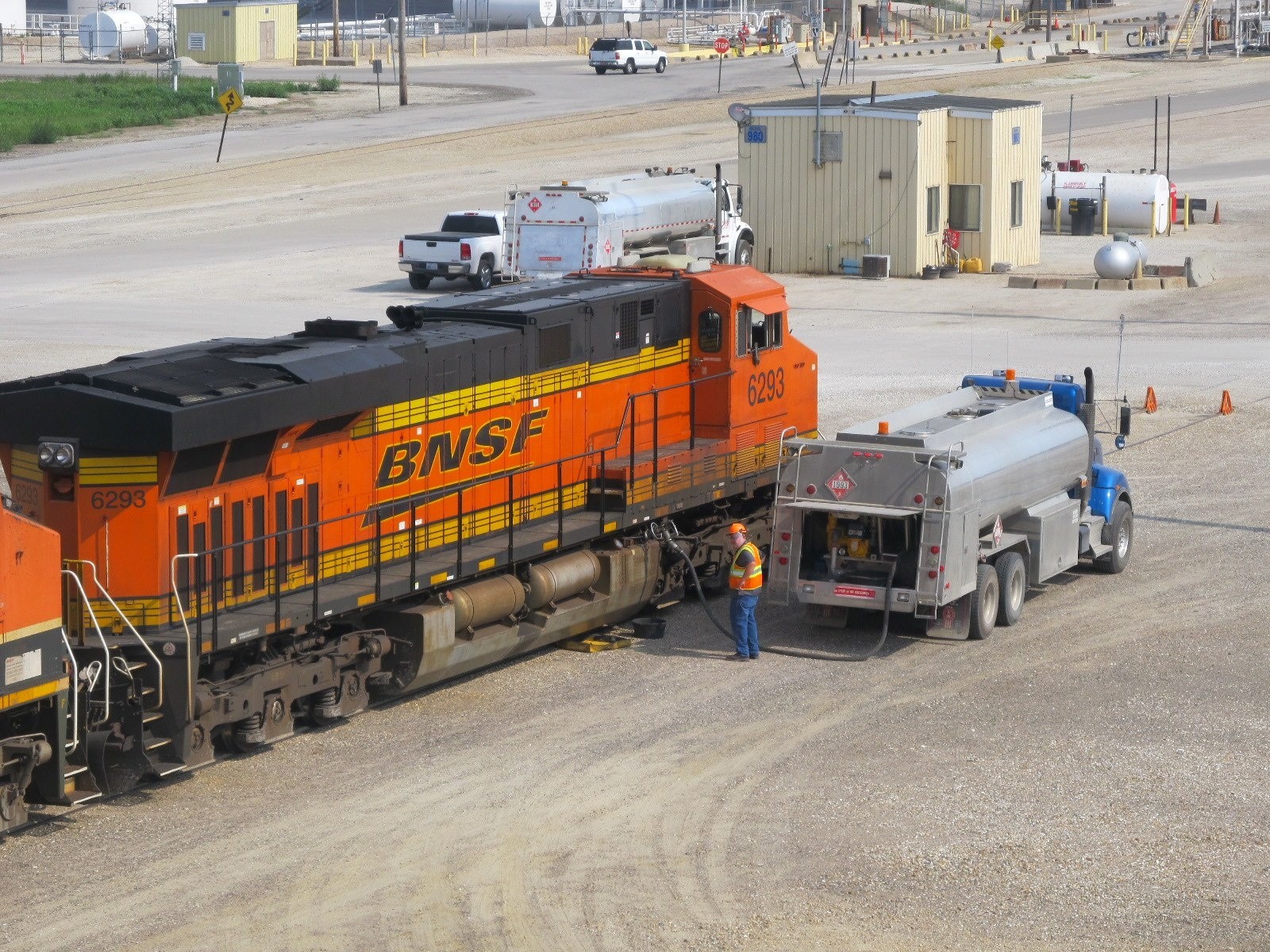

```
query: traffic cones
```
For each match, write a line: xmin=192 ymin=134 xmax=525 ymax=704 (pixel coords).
xmin=1220 ymin=390 xmax=1233 ymax=415
xmin=1144 ymin=385 xmax=1157 ymax=414
xmin=1054 ymin=16 xmax=1059 ymax=29
xmin=987 ymin=20 xmax=993 ymax=28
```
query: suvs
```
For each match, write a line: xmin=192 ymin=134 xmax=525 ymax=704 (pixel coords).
xmin=588 ymin=37 xmax=669 ymax=74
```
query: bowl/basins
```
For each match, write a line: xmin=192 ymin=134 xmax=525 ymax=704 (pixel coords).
xmin=922 ymin=264 xmax=958 ymax=280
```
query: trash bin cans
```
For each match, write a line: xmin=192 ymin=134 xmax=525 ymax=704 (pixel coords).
xmin=1157 ymin=12 xmax=1166 ymax=24
xmin=1069 ymin=198 xmax=1098 ymax=236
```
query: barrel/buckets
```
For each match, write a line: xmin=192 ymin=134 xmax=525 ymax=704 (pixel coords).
xmin=950 ymin=257 xmax=982 ymax=273
xmin=1069 ymin=197 xmax=1098 ymax=237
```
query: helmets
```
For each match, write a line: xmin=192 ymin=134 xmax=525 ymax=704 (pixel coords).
xmin=725 ymin=523 xmax=747 ymax=536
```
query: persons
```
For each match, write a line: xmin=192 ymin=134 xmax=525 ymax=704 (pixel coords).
xmin=726 ymin=523 xmax=766 ymax=662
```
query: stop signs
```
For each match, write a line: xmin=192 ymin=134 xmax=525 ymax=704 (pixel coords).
xmin=715 ymin=38 xmax=730 ymax=54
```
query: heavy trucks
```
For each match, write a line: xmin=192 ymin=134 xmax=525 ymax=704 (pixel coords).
xmin=766 ymin=369 xmax=1135 ymax=642
xmin=501 ymin=167 xmax=755 ymax=285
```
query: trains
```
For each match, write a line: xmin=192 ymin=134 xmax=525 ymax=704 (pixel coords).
xmin=0 ymin=262 xmax=821 ymax=839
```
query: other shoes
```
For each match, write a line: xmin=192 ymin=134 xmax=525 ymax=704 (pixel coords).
xmin=726 ymin=652 xmax=751 ymax=661
xmin=750 ymin=655 xmax=760 ymax=660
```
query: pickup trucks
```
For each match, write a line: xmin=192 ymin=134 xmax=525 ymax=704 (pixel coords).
xmin=398 ymin=210 xmax=507 ymax=289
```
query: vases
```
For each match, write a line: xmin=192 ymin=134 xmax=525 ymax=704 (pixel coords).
xmin=923 ymin=266 xmax=959 ymax=280
